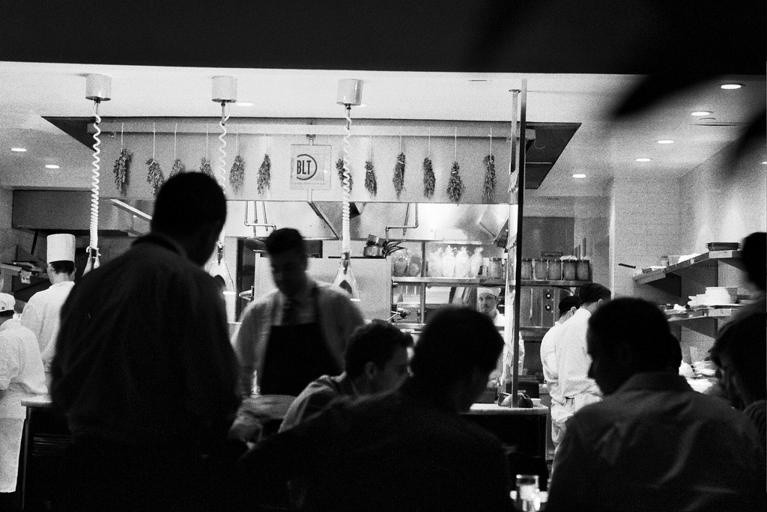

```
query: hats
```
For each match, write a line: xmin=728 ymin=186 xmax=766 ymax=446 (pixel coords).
xmin=477 ymin=286 xmax=501 ymax=298
xmin=46 ymin=234 xmax=76 ymax=263
xmin=0 ymin=293 xmax=15 ymax=312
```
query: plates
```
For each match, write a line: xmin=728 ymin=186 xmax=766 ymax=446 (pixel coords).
xmin=705 ymin=286 xmax=738 ymax=305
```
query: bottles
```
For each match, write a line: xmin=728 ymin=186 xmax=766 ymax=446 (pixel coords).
xmin=488 ymin=256 xmax=503 ymax=279
xmin=521 ymin=258 xmax=591 ymax=282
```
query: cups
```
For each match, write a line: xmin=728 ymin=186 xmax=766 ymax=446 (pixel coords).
xmin=514 ymin=474 xmax=541 ymax=512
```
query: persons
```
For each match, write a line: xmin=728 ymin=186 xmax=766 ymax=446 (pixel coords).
xmin=734 ymin=233 xmax=766 ymax=303
xmin=706 ymin=314 xmax=766 ymax=445
xmin=538 ymin=296 xmax=582 ymax=447
xmin=702 ymin=326 xmax=753 ymax=413
xmin=560 ymin=282 xmax=613 ymax=434
xmin=246 ymin=308 xmax=518 ymax=509
xmin=230 ymin=227 xmax=363 ymax=441
xmin=0 ymin=291 xmax=52 ymax=510
xmin=17 ymin=261 xmax=78 ymax=398
xmin=281 ymin=318 xmax=413 ymax=442
xmin=546 ymin=296 xmax=766 ymax=509
xmin=49 ymin=170 xmax=242 ymax=510
xmin=475 ymin=285 xmax=525 ymax=402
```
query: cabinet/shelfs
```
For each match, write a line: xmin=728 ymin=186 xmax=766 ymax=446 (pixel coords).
xmin=633 ymin=244 xmax=756 ymax=324
xmin=389 ymin=277 xmax=593 ymax=343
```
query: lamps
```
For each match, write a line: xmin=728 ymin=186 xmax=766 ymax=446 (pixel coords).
xmin=82 ymin=70 xmax=110 ymax=279
xmin=332 ymin=75 xmax=363 ymax=304
xmin=210 ymin=73 xmax=238 ymax=295
